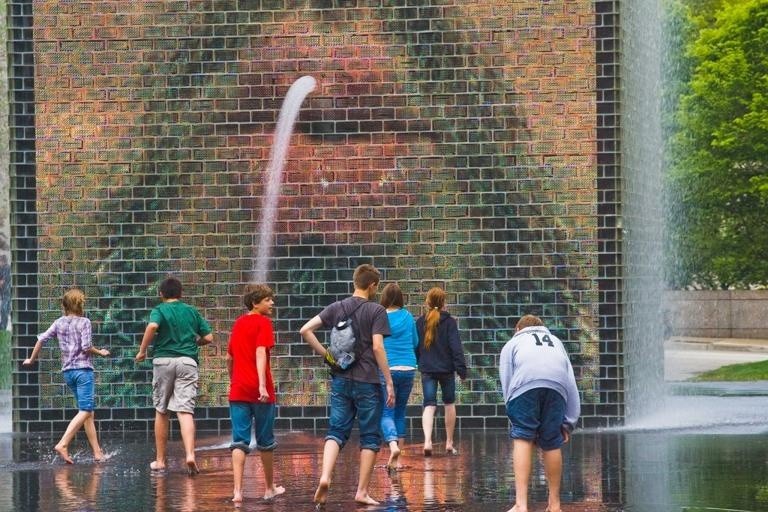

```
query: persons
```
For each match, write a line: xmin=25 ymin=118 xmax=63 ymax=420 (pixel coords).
xmin=51 ymin=467 xmax=107 ymax=512
xmin=23 ymin=288 xmax=112 ymax=462
xmin=379 ymin=466 xmax=410 ymax=511
xmin=149 ymin=472 xmax=198 ymax=512
xmin=372 ymin=282 xmax=421 ymax=470
xmin=226 ymin=280 xmax=289 ymax=504
xmin=418 ymin=458 xmax=466 ymax=508
xmin=497 ymin=314 xmax=584 ymax=511
xmin=134 ymin=278 xmax=216 ymax=475
xmin=297 ymin=264 xmax=399 ymax=506
xmin=415 ymin=287 xmax=469 ymax=457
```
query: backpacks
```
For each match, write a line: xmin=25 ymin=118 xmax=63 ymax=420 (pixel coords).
xmin=330 ymin=299 xmax=372 ymax=370
